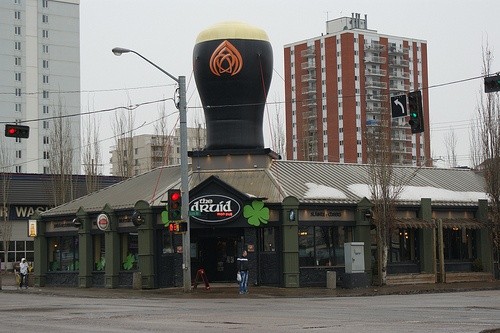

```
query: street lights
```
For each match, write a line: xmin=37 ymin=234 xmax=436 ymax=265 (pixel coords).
xmin=111 ymin=47 xmax=192 ymax=293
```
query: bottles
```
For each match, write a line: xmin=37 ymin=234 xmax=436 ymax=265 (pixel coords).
xmin=316 ymin=258 xmax=319 ymax=266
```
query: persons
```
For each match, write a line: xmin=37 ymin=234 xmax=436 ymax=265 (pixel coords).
xmin=19 ymin=258 xmax=30 ymax=289
xmin=235 ymin=248 xmax=250 ymax=296
xmin=192 ymin=253 xmax=210 ymax=290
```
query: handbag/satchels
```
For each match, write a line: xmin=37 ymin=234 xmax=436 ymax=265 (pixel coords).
xmin=237 ymin=273 xmax=241 ymax=281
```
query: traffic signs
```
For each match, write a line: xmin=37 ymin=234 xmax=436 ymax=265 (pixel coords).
xmin=390 ymin=95 xmax=407 ymax=119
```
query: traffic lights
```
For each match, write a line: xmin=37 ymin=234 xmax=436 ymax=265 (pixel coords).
xmin=168 ymin=221 xmax=187 ymax=233
xmin=5 ymin=124 xmax=30 ymax=139
xmin=407 ymin=90 xmax=424 ymax=134
xmin=484 ymin=76 xmax=500 ymax=93
xmin=168 ymin=189 xmax=181 ymax=221
